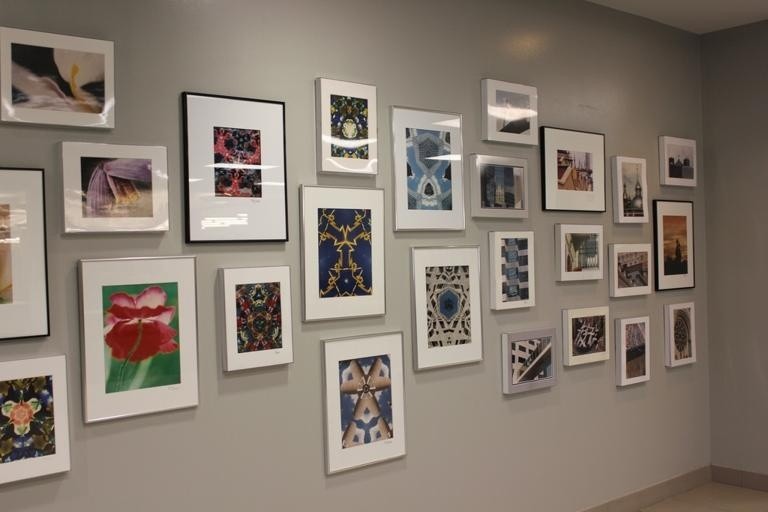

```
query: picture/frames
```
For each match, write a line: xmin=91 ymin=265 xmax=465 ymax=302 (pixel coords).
xmin=321 ymin=331 xmax=406 ymax=476
xmin=58 ymin=141 xmax=169 ymax=234
xmin=488 ymin=231 xmax=536 ymax=310
xmin=501 ymin=328 xmax=560 ymax=395
xmin=540 ymin=125 xmax=606 ymax=213
xmin=0 ymin=355 xmax=71 ymax=484
xmin=554 ymin=223 xmax=605 ymax=282
xmin=481 ymin=78 xmax=539 ymax=147
xmin=0 ymin=26 xmax=116 ymax=130
xmin=653 ymin=200 xmax=695 ymax=291
xmin=0 ymin=166 xmax=50 ymax=338
xmin=181 ymin=92 xmax=288 ymax=243
xmin=663 ymin=302 xmax=696 ymax=368
xmin=299 ymin=184 xmax=386 ymax=324
xmin=562 ymin=306 xmax=610 ymax=367
xmin=615 ymin=316 xmax=651 ymax=386
xmin=79 ymin=254 xmax=200 ymax=423
xmin=609 ymin=244 xmax=652 ymax=297
xmin=658 ymin=135 xmax=698 ymax=187
xmin=470 ymin=153 xmax=528 ymax=218
xmin=611 ymin=156 xmax=649 ymax=224
xmin=314 ymin=78 xmax=377 ymax=176
xmin=408 ymin=245 xmax=484 ymax=372
xmin=218 ymin=266 xmax=293 ymax=371
xmin=389 ymin=103 xmax=465 ymax=230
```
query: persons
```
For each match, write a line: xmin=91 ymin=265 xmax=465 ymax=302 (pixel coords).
xmin=675 ymin=239 xmax=682 ymax=262
